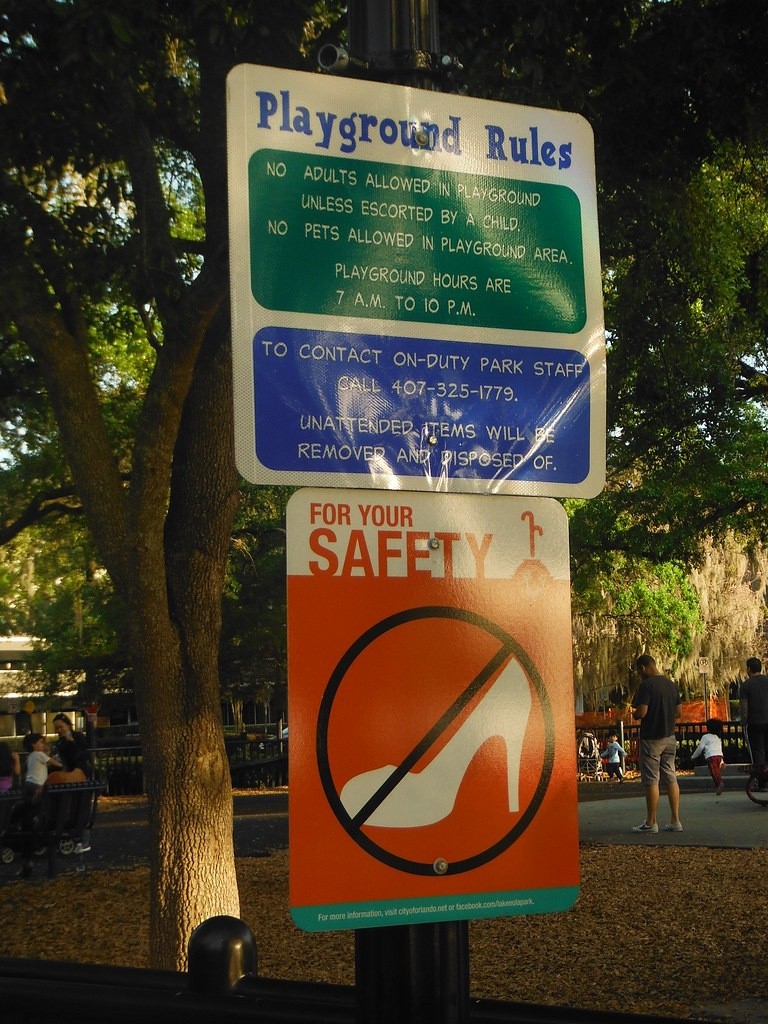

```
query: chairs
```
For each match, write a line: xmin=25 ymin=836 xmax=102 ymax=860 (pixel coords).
xmin=8 ymin=781 xmax=109 ymax=878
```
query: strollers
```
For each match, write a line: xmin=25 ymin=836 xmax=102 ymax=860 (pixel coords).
xmin=577 ymin=732 xmax=603 ymax=782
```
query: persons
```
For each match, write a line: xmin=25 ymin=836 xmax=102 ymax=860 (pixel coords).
xmin=631 ymin=655 xmax=683 ymax=832
xmin=0 ymin=713 xmax=92 ymax=853
xmin=601 ymin=731 xmax=627 ymax=784
xmin=22 ymin=733 xmax=63 ymax=803
xmin=739 ymin=657 xmax=768 ymax=791
xmin=690 ymin=718 xmax=726 ymax=795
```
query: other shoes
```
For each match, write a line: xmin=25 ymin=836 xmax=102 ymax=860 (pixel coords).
xmin=620 ymin=778 xmax=623 ymax=784
xmin=716 ymin=783 xmax=724 ymax=795
xmin=72 ymin=843 xmax=92 ymax=853
xmin=608 ymin=778 xmax=614 ymax=782
xmin=751 ymin=787 xmax=767 ymax=792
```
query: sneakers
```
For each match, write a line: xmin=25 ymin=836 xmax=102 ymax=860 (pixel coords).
xmin=632 ymin=820 xmax=658 ymax=834
xmin=664 ymin=822 xmax=683 ymax=832
